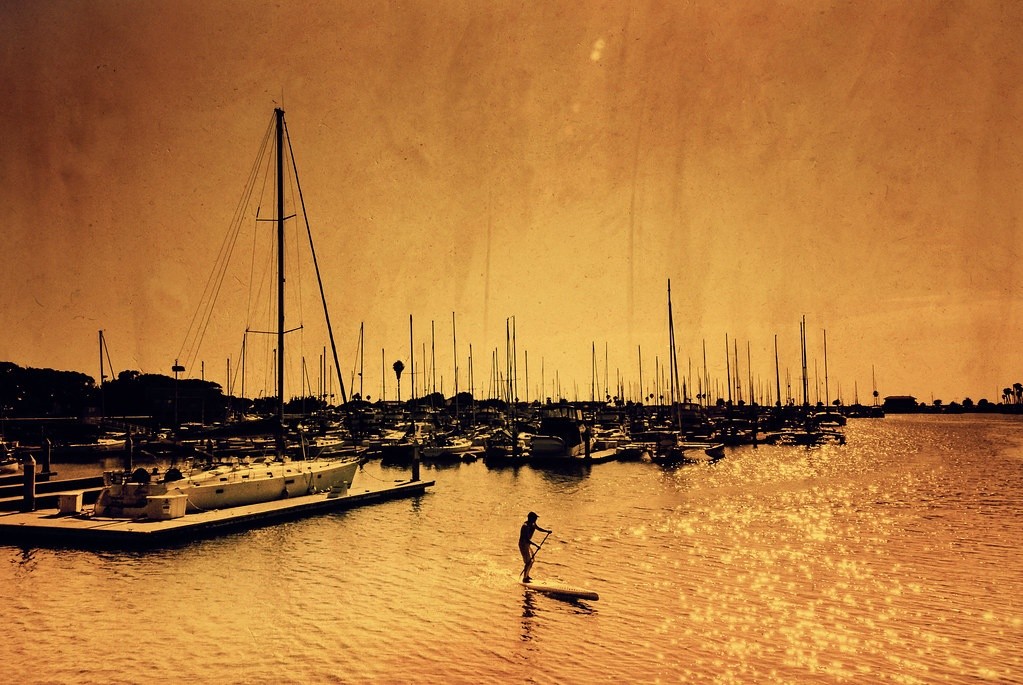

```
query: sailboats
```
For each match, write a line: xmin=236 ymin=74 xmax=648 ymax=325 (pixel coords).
xmin=96 ymin=88 xmax=371 ymax=518
xmin=95 ymin=278 xmax=886 ymax=463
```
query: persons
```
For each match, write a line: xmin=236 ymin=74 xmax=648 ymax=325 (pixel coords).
xmin=519 ymin=512 xmax=552 ymax=582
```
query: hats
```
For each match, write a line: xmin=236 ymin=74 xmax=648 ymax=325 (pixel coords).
xmin=527 ymin=512 xmax=539 ymax=517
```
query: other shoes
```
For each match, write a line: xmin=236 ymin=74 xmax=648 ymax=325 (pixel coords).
xmin=523 ymin=576 xmax=532 ymax=583
